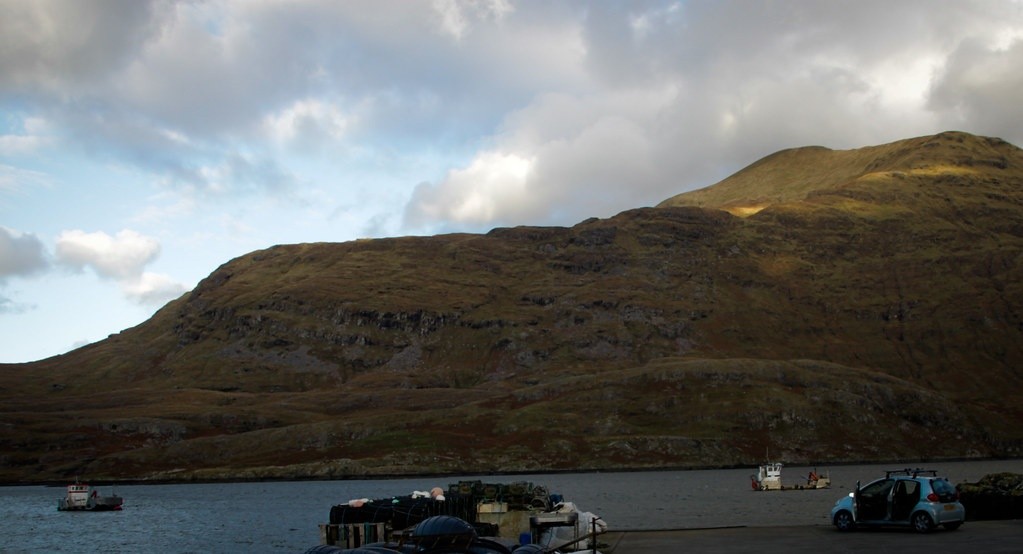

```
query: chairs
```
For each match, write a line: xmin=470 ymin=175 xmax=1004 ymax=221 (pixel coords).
xmin=895 ymin=482 xmax=907 ymax=498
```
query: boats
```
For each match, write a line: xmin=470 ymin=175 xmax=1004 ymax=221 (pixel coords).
xmin=56 ymin=485 xmax=123 ymax=511
xmin=751 ymin=446 xmax=830 ymax=491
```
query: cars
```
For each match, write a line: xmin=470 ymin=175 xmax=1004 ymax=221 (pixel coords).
xmin=831 ymin=467 xmax=965 ymax=535
